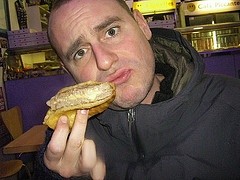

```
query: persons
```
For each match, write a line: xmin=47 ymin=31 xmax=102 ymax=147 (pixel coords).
xmin=35 ymin=0 xmax=240 ymax=180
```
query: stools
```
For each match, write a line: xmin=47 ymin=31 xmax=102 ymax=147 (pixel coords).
xmin=0 ymin=159 xmax=31 ymax=180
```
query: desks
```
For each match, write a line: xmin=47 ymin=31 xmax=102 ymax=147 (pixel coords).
xmin=2 ymin=125 xmax=49 ymax=180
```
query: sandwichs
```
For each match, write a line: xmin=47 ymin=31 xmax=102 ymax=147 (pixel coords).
xmin=42 ymin=81 xmax=116 ymax=130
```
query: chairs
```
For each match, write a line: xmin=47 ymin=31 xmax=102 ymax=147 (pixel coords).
xmin=0 ymin=106 xmax=23 ymax=140
